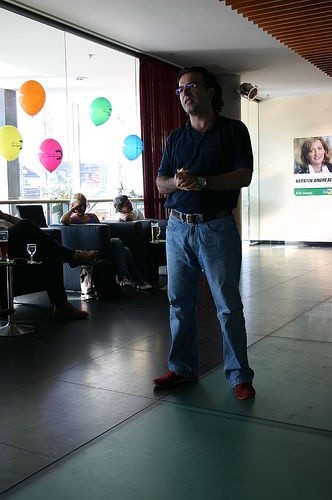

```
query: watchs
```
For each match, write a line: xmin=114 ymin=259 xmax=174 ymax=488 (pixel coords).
xmin=195 ymin=175 xmax=208 ymax=190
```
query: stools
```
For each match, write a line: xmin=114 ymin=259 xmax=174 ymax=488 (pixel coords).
xmin=0 ymin=260 xmax=35 ymax=336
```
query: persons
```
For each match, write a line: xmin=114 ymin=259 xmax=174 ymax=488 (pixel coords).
xmin=60 ymin=193 xmax=152 ymax=290
xmin=153 ymin=66 xmax=255 ymax=401
xmin=294 ymin=137 xmax=332 ymax=174
xmin=0 ymin=209 xmax=99 ymax=319
xmin=113 ymin=195 xmax=150 ymax=221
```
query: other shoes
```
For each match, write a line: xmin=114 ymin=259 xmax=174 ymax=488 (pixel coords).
xmin=54 ymin=302 xmax=88 ymax=320
xmin=69 ymin=250 xmax=102 ymax=268
xmin=136 ymin=279 xmax=152 ymax=289
xmin=115 ymin=273 xmax=133 ymax=287
xmin=235 ymin=384 xmax=257 ymax=398
xmin=152 ymin=370 xmax=198 ymax=388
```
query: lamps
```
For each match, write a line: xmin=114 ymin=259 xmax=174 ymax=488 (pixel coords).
xmin=234 ymin=82 xmax=262 ymax=104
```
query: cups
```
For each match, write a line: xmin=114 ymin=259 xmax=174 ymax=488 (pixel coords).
xmin=151 ymin=223 xmax=161 ymax=242
xmin=0 ymin=230 xmax=10 ymax=261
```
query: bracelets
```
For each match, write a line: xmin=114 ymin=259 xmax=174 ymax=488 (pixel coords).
xmin=70 ymin=207 xmax=77 ymax=213
xmin=6 ymin=215 xmax=11 ymax=221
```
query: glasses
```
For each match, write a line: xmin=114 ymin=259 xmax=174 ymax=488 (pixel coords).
xmin=125 ymin=200 xmax=132 ymax=207
xmin=176 ymin=82 xmax=204 ymax=96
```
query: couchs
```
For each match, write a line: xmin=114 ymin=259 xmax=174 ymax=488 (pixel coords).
xmin=47 ymin=218 xmax=166 ymax=291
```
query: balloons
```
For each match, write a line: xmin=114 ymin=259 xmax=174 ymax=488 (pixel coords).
xmin=0 ymin=125 xmax=23 ymax=162
xmin=89 ymin=96 xmax=112 ymax=127
xmin=122 ymin=134 xmax=144 ymax=160
xmin=38 ymin=138 xmax=64 ymax=173
xmin=19 ymin=80 xmax=47 ymax=117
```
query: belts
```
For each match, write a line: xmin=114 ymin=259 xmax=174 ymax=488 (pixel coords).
xmin=171 ymin=209 xmax=233 ymax=224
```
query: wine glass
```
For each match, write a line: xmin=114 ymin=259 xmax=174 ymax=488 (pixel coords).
xmin=27 ymin=244 xmax=36 ymax=263
